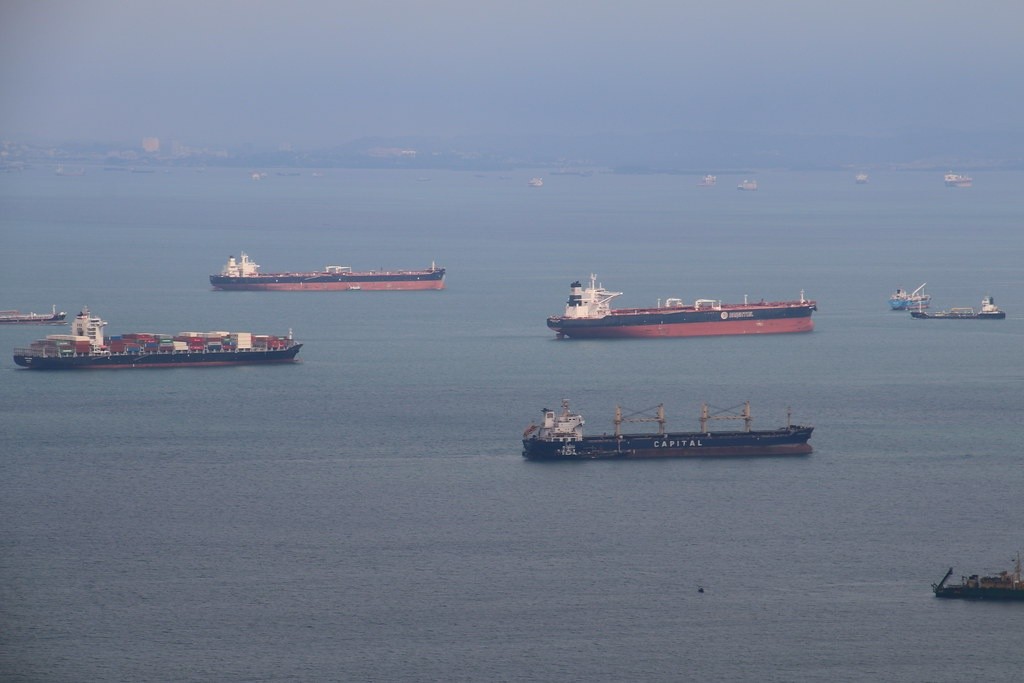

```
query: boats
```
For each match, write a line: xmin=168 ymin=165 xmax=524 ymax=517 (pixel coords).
xmin=888 ymin=279 xmax=932 ymax=311
xmin=855 ymin=170 xmax=869 ymax=185
xmin=734 ymin=176 xmax=758 ymax=192
xmin=523 ymin=396 xmax=816 ymax=460
xmin=944 ymin=171 xmax=973 ymax=188
xmin=12 ymin=307 xmax=305 ymax=369
xmin=905 ymin=296 xmax=1005 ymax=320
xmin=0 ymin=306 xmax=67 ymax=328
xmin=931 ymin=551 xmax=1024 ymax=600
xmin=544 ymin=272 xmax=820 ymax=338
xmin=528 ymin=176 xmax=544 ymax=189
xmin=696 ymin=173 xmax=718 ymax=186
xmin=210 ymin=252 xmax=447 ymax=293
